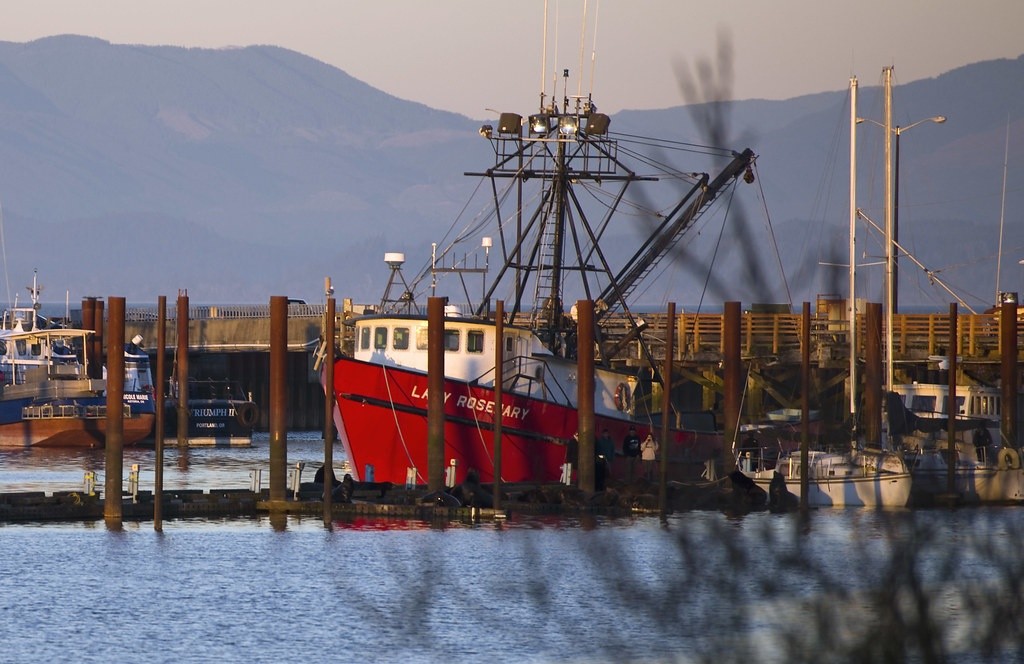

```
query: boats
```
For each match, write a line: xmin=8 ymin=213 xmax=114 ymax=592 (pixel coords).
xmin=0 ymin=270 xmax=156 ymax=446
xmin=163 ymin=289 xmax=260 ymax=448
xmin=727 ymin=384 xmax=1024 ymax=508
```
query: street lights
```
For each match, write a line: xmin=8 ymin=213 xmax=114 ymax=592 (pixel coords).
xmin=855 ymin=116 xmax=945 ymax=391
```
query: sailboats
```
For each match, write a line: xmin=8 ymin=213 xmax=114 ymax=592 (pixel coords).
xmin=312 ymin=65 xmax=825 ymax=486
